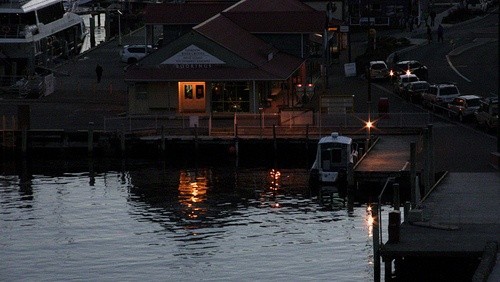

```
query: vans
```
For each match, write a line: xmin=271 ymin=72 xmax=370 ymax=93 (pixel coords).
xmin=420 ymin=83 xmax=460 ymax=109
xmin=119 ymin=45 xmax=159 ymax=66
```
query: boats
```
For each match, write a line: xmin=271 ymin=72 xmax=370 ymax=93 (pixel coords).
xmin=305 ymin=132 xmax=359 ymax=197
xmin=0 ymin=0 xmax=89 ymax=86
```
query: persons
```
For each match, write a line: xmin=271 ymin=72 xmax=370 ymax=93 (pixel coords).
xmin=437 ymin=23 xmax=445 ymax=43
xmin=95 ymin=63 xmax=104 ymax=83
xmin=393 ymin=53 xmax=399 ymax=63
xmin=460 ymin=0 xmax=488 ymax=13
xmin=426 ymin=24 xmax=433 ymax=46
xmin=430 ymin=10 xmax=437 ymax=27
xmin=398 ymin=12 xmax=419 ymax=33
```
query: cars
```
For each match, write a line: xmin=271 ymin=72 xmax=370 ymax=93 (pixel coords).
xmin=389 ymin=61 xmax=429 ymax=103
xmin=365 ymin=61 xmax=391 ymax=82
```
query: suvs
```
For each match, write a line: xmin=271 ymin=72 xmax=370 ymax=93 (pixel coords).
xmin=445 ymin=93 xmax=499 ymax=132
